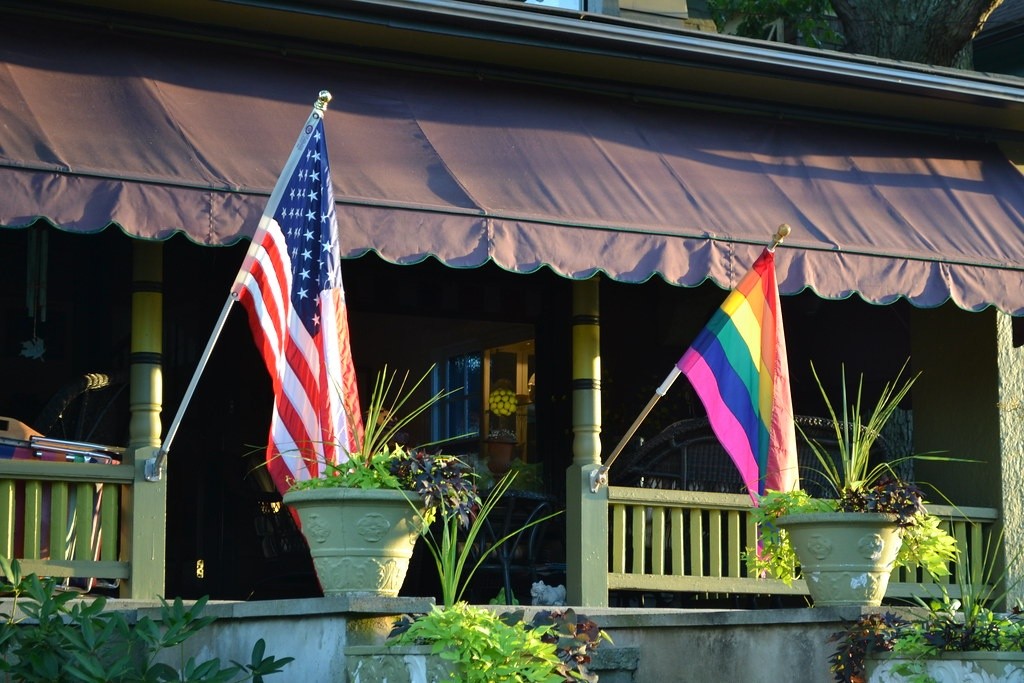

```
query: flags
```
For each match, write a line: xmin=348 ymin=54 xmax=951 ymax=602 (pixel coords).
xmin=229 ymin=114 xmax=363 ymax=544
xmin=680 ymin=249 xmax=800 ymax=576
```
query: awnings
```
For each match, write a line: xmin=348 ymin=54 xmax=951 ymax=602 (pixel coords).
xmin=0 ymin=25 xmax=1024 ymax=317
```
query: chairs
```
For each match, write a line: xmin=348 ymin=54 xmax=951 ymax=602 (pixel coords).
xmin=244 ymin=486 xmax=322 ymax=597
xmin=29 ymin=369 xmax=131 ymax=452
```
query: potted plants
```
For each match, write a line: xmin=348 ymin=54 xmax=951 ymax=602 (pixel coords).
xmin=281 ymin=361 xmax=486 ymax=598
xmin=737 ymin=352 xmax=964 ymax=608
xmin=340 ymin=604 xmax=617 ymax=683
xmin=826 ymin=522 xmax=1023 ymax=683
xmin=484 ymin=429 xmax=520 ymax=471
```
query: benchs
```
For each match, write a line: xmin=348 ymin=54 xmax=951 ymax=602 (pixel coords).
xmin=608 ymin=410 xmax=898 ymax=502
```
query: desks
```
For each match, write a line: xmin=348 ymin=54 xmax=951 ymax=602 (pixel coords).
xmin=461 ymin=486 xmax=564 ymax=606
